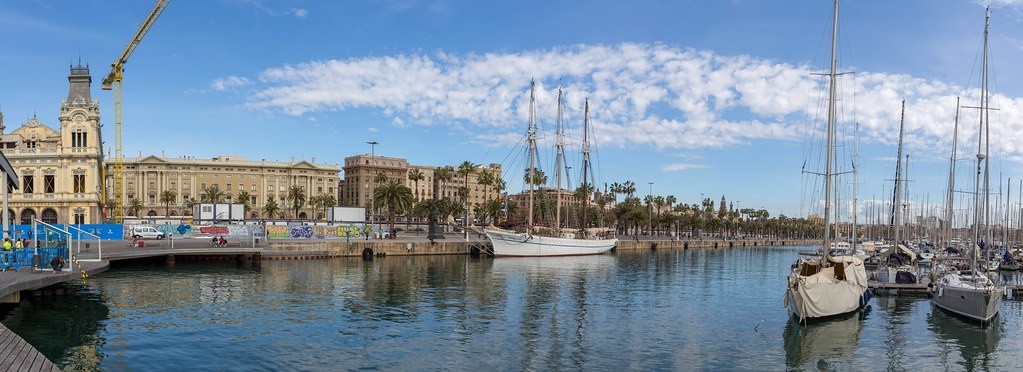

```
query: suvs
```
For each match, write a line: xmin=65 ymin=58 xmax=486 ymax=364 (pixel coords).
xmin=130 ymin=227 xmax=165 ymax=240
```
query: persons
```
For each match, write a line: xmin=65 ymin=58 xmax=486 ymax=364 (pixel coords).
xmin=28 ymin=229 xmax=32 ymax=239
xmin=16 ymin=238 xmax=24 ymax=248
xmin=220 ymin=236 xmax=225 ymax=247
xmin=23 ymin=238 xmax=30 ymax=248
xmin=94 ymin=228 xmax=96 ymax=235
xmin=688 ymin=233 xmax=690 ymax=240
xmin=378 ymin=228 xmax=382 ymax=240
xmin=366 ymin=230 xmax=369 ymax=239
xmin=682 ymin=236 xmax=684 ymax=240
xmin=51 ymin=256 xmax=63 ymax=273
xmin=3 ymin=236 xmax=12 ymax=268
xmin=633 ymin=235 xmax=637 ymax=240
xmin=37 ymin=237 xmax=40 ymax=251
xmin=213 ymin=236 xmax=218 ymax=248
xmin=56 ymin=237 xmax=67 ymax=248
xmin=48 ymin=231 xmax=54 ymax=244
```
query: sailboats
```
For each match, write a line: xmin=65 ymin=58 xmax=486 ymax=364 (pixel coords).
xmin=444 ymin=75 xmax=619 ymax=258
xmin=784 ymin=0 xmax=873 ymax=326
xmin=830 ymin=2 xmax=1023 ymax=328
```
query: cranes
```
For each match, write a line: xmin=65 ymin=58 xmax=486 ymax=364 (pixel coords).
xmin=100 ymin=0 xmax=168 ymax=224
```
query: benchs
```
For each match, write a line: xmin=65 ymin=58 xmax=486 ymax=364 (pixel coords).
xmin=208 ymin=241 xmax=242 ymax=248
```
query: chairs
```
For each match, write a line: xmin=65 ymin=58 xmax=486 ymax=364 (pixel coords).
xmin=31 ymin=254 xmax=43 ymax=272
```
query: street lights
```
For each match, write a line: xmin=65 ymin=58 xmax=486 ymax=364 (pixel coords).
xmin=736 ymin=200 xmax=741 ymax=236
xmin=366 ymin=141 xmax=379 ymax=226
xmin=648 ymin=182 xmax=654 ymax=236
xmin=701 ymin=193 xmax=705 ymax=208
